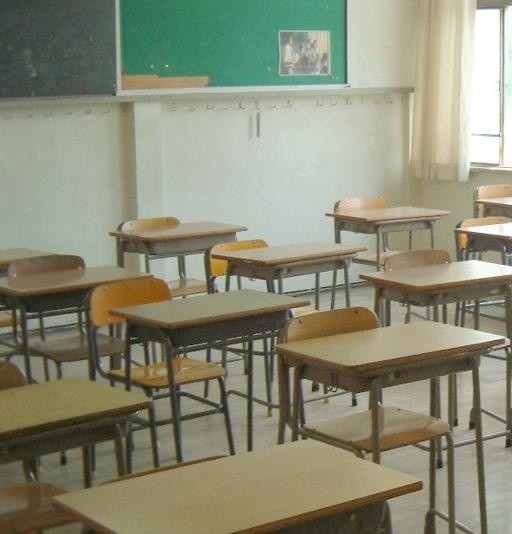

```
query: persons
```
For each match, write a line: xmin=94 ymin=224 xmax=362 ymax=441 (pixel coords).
xmin=281 ymin=31 xmax=329 ymax=74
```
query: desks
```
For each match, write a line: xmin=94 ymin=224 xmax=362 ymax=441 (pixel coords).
xmin=0 ymin=381 xmax=154 ymax=487
xmin=276 ymin=320 xmax=510 ymax=470
xmin=54 ymin=441 xmax=426 ymax=533
xmin=0 ymin=198 xmax=512 ymax=465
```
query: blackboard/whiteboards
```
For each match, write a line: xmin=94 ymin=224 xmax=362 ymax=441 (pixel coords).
xmin=119 ymin=4 xmax=349 ymax=89
xmin=2 ymin=3 xmax=117 ymax=98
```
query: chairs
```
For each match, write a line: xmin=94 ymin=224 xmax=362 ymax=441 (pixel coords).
xmin=473 ymin=185 xmax=512 ymax=218
xmin=3 ymin=488 xmax=80 ymax=534
